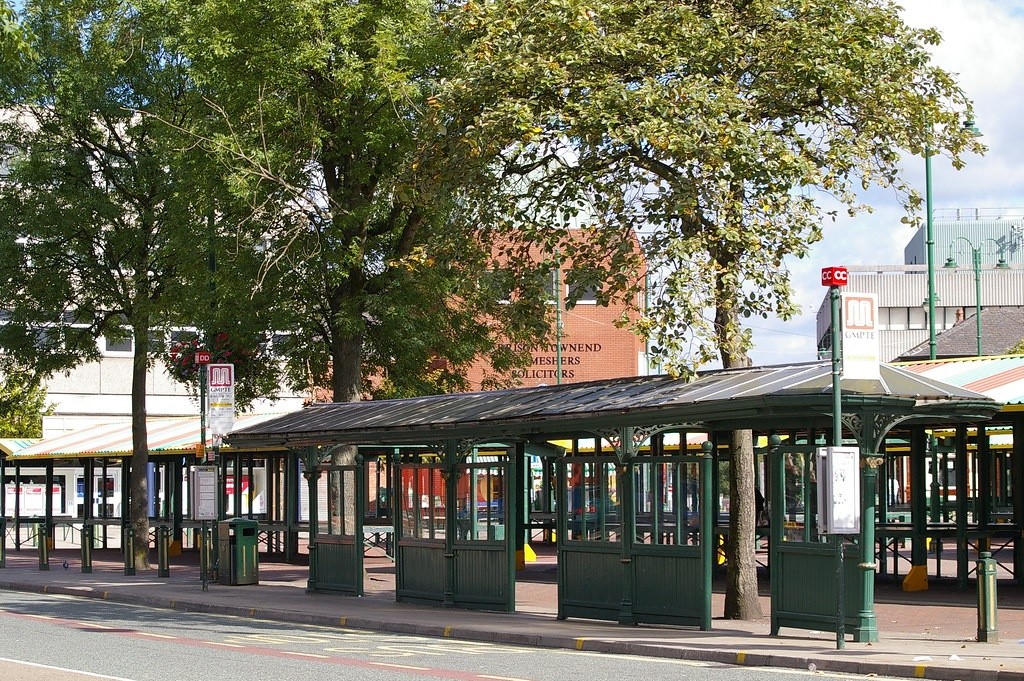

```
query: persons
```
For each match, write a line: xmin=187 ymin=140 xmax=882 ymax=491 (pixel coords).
xmin=754 ymin=486 xmax=764 ymax=526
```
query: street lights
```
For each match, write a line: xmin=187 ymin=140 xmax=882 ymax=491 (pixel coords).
xmin=943 ymin=236 xmax=1011 ymax=357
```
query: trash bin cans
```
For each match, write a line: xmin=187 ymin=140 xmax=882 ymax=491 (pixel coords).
xmin=218 ymin=517 xmax=260 ymax=585
